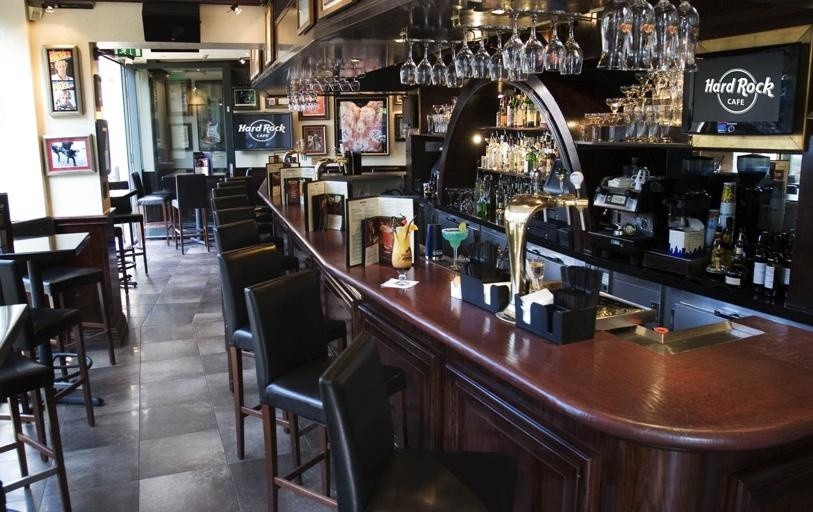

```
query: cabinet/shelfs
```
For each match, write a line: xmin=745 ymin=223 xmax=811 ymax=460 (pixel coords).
xmin=412 ymin=53 xmax=690 ymax=232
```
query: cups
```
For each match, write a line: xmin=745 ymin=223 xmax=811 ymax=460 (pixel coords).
xmin=596 ymin=0 xmax=698 ymax=71
xmin=630 ymin=169 xmax=650 ymax=191
xmin=424 ymin=96 xmax=458 ymax=135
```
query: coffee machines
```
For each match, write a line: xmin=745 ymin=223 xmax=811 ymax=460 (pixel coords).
xmin=734 ymin=154 xmax=771 ymax=232
xmin=587 ymin=176 xmax=667 ymax=266
xmin=678 ymin=155 xmax=714 ymax=220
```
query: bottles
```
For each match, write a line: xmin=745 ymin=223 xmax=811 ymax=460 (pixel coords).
xmin=717 ymin=180 xmax=737 ymax=239
xmin=424 ymin=223 xmax=444 ymax=261
xmin=391 ymin=226 xmax=412 ymax=270
xmin=703 ymin=210 xmax=718 ymax=247
xmin=710 ymin=216 xmax=796 ymax=300
xmin=494 ymin=94 xmax=548 ymax=130
xmin=478 ymin=130 xmax=557 ymax=220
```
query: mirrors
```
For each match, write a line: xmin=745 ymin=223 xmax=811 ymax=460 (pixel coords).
xmin=691 ymin=149 xmax=801 ymax=285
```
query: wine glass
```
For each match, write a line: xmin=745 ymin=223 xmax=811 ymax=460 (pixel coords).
xmin=288 ymin=58 xmax=361 ymax=112
xmin=523 ymin=257 xmax=544 ymax=292
xmin=582 ymin=70 xmax=681 ymax=129
xmin=440 ymin=228 xmax=469 ymax=270
xmin=444 ymin=187 xmax=472 ymax=212
xmin=398 ymin=6 xmax=583 ymax=89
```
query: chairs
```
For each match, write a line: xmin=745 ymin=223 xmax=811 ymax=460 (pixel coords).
xmin=138 ymin=162 xmax=262 ymax=254
xmin=0 ymin=181 xmax=147 ymax=512
xmin=319 ymin=323 xmax=522 ymax=511
xmin=217 ymin=243 xmax=349 ymax=460
xmin=242 ymin=263 xmax=402 ymax=512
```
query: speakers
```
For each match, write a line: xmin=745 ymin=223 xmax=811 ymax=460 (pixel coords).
xmin=141 ymin=0 xmax=201 ymax=42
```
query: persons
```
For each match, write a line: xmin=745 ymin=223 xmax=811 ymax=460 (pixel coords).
xmin=54 ymin=88 xmax=75 ymax=110
xmin=52 ymin=60 xmax=74 ymax=80
xmin=51 ymin=142 xmax=77 ymax=167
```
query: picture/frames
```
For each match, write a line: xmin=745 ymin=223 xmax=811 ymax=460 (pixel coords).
xmin=41 ymin=44 xmax=84 ymax=119
xmin=391 ymin=94 xmax=401 ymax=106
xmin=163 ymin=78 xmax=194 ymax=117
xmin=333 ymin=96 xmax=389 ymax=157
xmin=393 ymin=113 xmax=405 ymax=142
xmin=301 ymin=125 xmax=327 ymax=157
xmin=316 ymin=0 xmax=353 ymax=23
xmin=168 ymin=123 xmax=193 ymax=151
xmin=297 ymin=94 xmax=328 ymax=121
xmin=295 ymin=0 xmax=316 ymax=37
xmin=41 ymin=134 xmax=95 ymax=178
xmin=231 ymin=86 xmax=260 ymax=111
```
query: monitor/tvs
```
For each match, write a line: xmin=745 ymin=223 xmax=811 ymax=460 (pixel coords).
xmin=681 ymin=42 xmax=801 ymax=133
xmin=232 ymin=112 xmax=294 ymax=150
xmin=234 ymin=89 xmax=256 ymax=106
xmin=542 ymin=158 xmax=568 ymax=195
